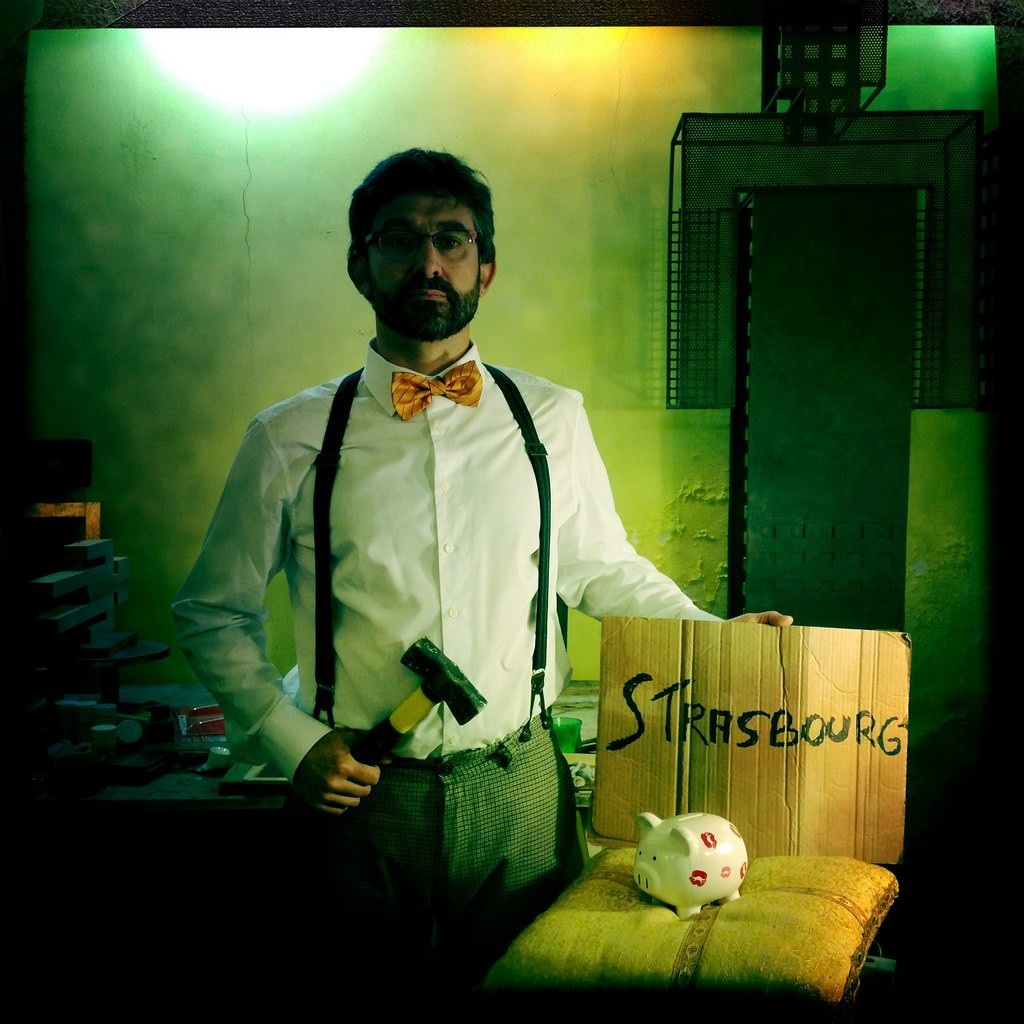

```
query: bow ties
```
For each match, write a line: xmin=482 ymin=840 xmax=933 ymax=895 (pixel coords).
xmin=392 ymin=361 xmax=485 ymax=421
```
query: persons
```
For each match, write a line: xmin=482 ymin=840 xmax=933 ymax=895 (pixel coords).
xmin=169 ymin=149 xmax=794 ymax=1024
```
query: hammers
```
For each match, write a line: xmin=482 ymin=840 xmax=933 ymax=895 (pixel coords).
xmin=348 ymin=635 xmax=488 ymax=767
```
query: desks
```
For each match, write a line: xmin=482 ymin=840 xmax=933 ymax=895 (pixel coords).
xmin=0 ymin=681 xmax=604 ymax=807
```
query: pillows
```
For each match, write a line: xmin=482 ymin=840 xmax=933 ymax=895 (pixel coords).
xmin=482 ymin=844 xmax=901 ymax=1008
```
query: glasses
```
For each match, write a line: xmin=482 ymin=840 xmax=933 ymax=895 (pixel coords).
xmin=363 ymin=229 xmax=477 ymax=259
